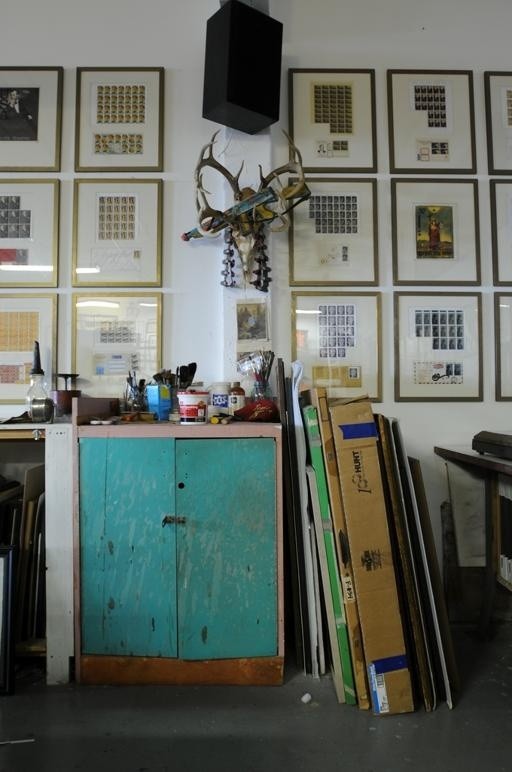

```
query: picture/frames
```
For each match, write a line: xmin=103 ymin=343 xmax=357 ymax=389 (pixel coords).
xmin=291 ymin=291 xmax=383 ymax=403
xmin=494 ymin=291 xmax=512 ymax=402
xmin=288 ymin=177 xmax=379 ymax=287
xmin=393 ymin=291 xmax=484 ymax=403
xmin=390 ymin=177 xmax=481 ymax=286
xmin=387 ymin=68 xmax=477 ymax=175
xmin=233 ymin=297 xmax=272 ymax=353
xmin=287 ymin=67 xmax=377 ymax=173
xmin=484 ymin=70 xmax=512 ymax=175
xmin=489 ymin=178 xmax=512 ymax=286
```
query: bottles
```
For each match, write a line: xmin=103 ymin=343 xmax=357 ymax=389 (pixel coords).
xmin=252 ymin=380 xmax=273 ymax=420
xmin=27 ymin=339 xmax=51 ymax=422
xmin=206 ymin=382 xmax=232 ymax=421
xmin=227 ymin=382 xmax=246 ymax=416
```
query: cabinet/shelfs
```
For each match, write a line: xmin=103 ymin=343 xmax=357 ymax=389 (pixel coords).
xmin=74 ymin=426 xmax=284 ymax=685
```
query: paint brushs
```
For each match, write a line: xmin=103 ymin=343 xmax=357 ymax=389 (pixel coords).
xmin=246 ymin=349 xmax=275 ymax=400
xmin=126 ymin=368 xmax=146 ymax=410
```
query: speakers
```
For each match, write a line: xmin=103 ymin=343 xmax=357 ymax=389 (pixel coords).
xmin=200 ymin=1 xmax=284 ymax=136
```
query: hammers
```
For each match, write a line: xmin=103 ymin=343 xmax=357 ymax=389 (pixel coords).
xmin=218 ymin=413 xmax=243 ymax=424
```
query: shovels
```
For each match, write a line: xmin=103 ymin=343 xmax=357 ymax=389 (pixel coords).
xmin=180 ymin=366 xmax=188 ymax=388
xmin=188 ymin=363 xmax=196 ymax=386
